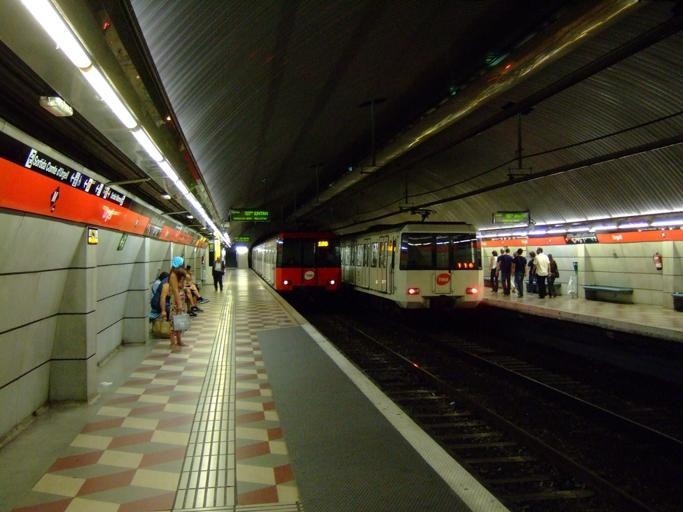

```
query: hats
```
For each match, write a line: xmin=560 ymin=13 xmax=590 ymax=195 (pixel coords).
xmin=171 ymin=256 xmax=184 ymax=269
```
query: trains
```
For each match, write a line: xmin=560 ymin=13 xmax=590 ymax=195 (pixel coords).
xmin=339 ymin=221 xmax=485 ymax=312
xmin=246 ymin=227 xmax=341 ymax=293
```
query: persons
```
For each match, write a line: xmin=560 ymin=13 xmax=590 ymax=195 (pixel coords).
xmin=488 ymin=247 xmax=560 ymax=299
xmin=149 ymin=256 xmax=209 ymax=351
xmin=212 ymin=255 xmax=225 ymax=291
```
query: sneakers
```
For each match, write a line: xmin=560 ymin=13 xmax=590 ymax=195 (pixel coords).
xmin=191 ymin=307 xmax=202 ymax=313
xmin=187 ymin=311 xmax=197 ymax=316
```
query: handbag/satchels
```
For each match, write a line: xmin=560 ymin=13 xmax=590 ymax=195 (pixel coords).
xmin=172 ymin=311 xmax=191 ymax=332
xmin=152 ymin=320 xmax=172 ymax=338
xmin=555 ymin=269 xmax=559 ymax=278
